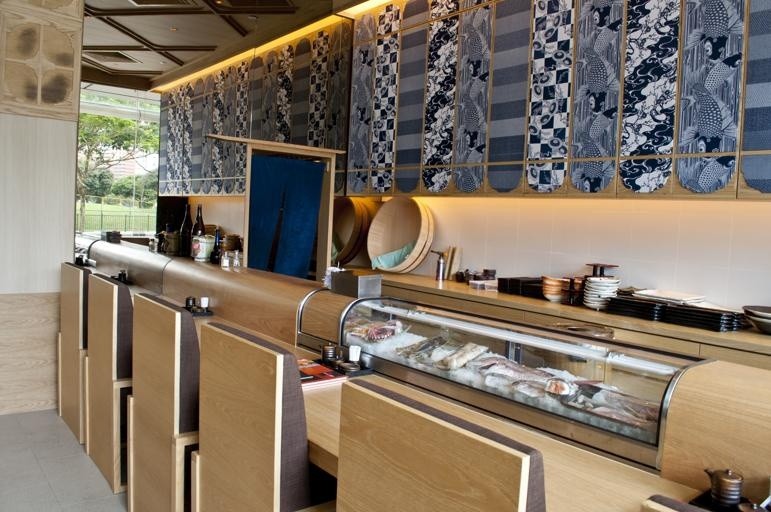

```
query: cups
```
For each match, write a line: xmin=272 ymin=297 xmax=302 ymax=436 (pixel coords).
xmin=319 ymin=340 xmax=337 ymax=363
xmin=185 ymin=295 xmax=195 ymax=309
xmin=118 ymin=273 xmax=126 ymax=281
xmin=704 ymin=468 xmax=770 ymax=512
xmin=465 ymin=268 xmax=496 ymax=285
xmin=75 ymin=257 xmax=83 ymax=266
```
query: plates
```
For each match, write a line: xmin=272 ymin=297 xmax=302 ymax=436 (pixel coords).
xmin=498 ymin=269 xmax=745 ymax=334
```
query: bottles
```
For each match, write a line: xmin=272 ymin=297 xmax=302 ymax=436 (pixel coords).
xmin=149 ymin=203 xmax=243 ymax=271
xmin=435 ymin=253 xmax=446 ymax=280
xmin=455 ymin=266 xmax=464 ymax=283
xmin=334 ymin=349 xmax=346 ymax=370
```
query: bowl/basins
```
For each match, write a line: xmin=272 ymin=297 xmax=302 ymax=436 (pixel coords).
xmin=366 ymin=196 xmax=435 ymax=280
xmin=743 ymin=305 xmax=771 ymax=332
xmin=330 ymin=196 xmax=367 ymax=266
